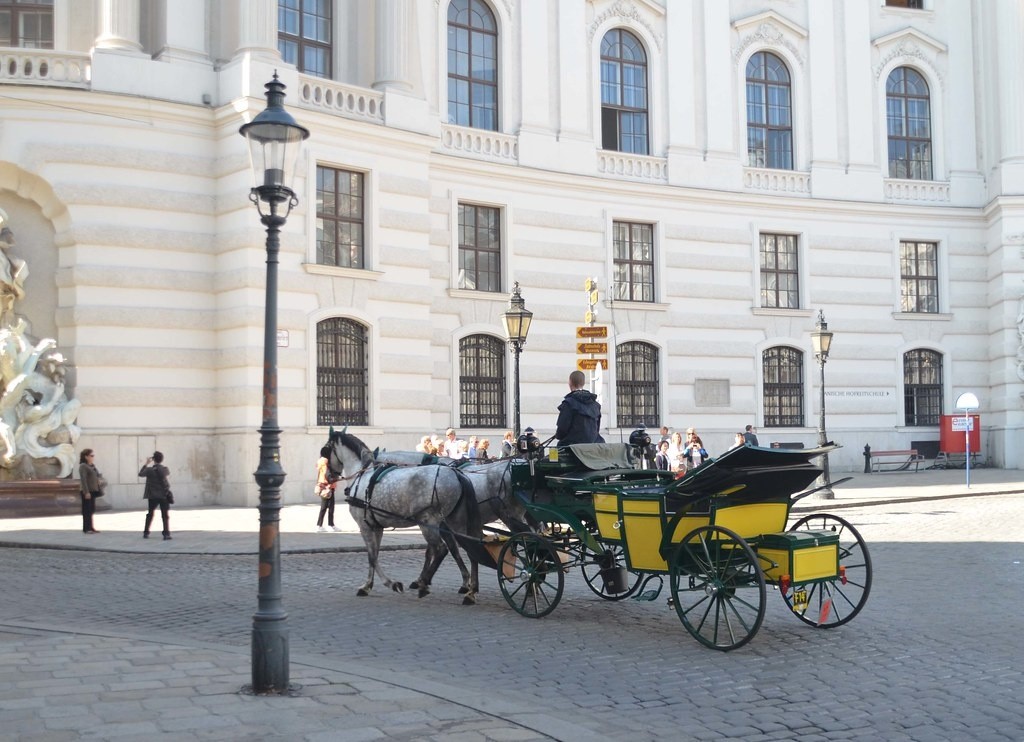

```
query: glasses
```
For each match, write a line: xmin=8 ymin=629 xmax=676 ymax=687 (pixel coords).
xmin=687 ymin=433 xmax=694 ymax=435
xmin=88 ymin=455 xmax=94 ymax=457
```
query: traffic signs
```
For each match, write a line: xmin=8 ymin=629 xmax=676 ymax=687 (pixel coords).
xmin=575 ymin=327 xmax=608 ymax=371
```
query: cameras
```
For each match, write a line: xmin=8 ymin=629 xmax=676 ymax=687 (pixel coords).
xmin=693 ymin=445 xmax=697 ymax=448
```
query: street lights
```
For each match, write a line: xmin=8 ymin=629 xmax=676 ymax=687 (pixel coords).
xmin=503 ymin=281 xmax=533 ymax=440
xmin=809 ymin=308 xmax=834 ymax=499
xmin=239 ymin=68 xmax=311 ymax=698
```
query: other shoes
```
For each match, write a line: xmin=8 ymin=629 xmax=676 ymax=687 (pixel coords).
xmin=315 ymin=526 xmax=328 ymax=532
xmin=327 ymin=526 xmax=341 ymax=532
xmin=164 ymin=535 xmax=172 ymax=540
xmin=83 ymin=528 xmax=100 ymax=534
xmin=143 ymin=534 xmax=148 ymax=539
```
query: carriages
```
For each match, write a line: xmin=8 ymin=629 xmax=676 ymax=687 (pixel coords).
xmin=321 ymin=423 xmax=874 ymax=651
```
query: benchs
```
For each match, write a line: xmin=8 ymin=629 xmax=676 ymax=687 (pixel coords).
xmin=870 ymin=449 xmax=926 ymax=475
xmin=511 ymin=442 xmax=631 ymax=491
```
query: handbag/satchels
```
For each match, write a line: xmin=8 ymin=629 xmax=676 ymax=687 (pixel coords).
xmin=167 ymin=490 xmax=174 ymax=504
xmin=94 ymin=470 xmax=107 ymax=497
xmin=314 ymin=482 xmax=332 ymax=498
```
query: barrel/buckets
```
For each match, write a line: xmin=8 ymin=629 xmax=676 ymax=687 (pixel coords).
xmin=599 ymin=564 xmax=628 ymax=594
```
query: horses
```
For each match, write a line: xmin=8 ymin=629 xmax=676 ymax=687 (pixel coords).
xmin=313 ymin=423 xmax=535 ymax=608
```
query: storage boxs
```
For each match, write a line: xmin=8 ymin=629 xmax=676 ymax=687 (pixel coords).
xmin=756 ymin=528 xmax=840 ymax=585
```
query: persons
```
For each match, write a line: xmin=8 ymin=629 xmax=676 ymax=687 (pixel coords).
xmin=139 ymin=451 xmax=175 ymax=540
xmin=475 ymin=439 xmax=497 ymax=461
xmin=468 ymin=435 xmax=479 ymax=459
xmin=669 ymin=432 xmax=686 ymax=475
xmin=416 ymin=435 xmax=437 ymax=457
xmin=317 ymin=456 xmax=342 ymax=532
xmin=682 ymin=437 xmax=708 ymax=472
xmin=654 ymin=440 xmax=672 ymax=486
xmin=553 ymin=371 xmax=605 ymax=448
xmin=79 ymin=449 xmax=106 ymax=534
xmin=443 ymin=429 xmax=464 ymax=459
xmin=500 ymin=431 xmax=515 ymax=460
xmin=744 ymin=424 xmax=759 ymax=447
xmin=658 ymin=427 xmax=672 ymax=443
xmin=728 ymin=432 xmax=744 ymax=451
xmin=457 ymin=440 xmax=471 ymax=459
xmin=773 ymin=441 xmax=780 ymax=449
xmin=431 ymin=434 xmax=450 ymax=458
xmin=684 ymin=427 xmax=704 ymax=449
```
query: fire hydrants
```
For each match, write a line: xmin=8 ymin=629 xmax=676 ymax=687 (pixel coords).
xmin=863 ymin=443 xmax=871 ymax=473
